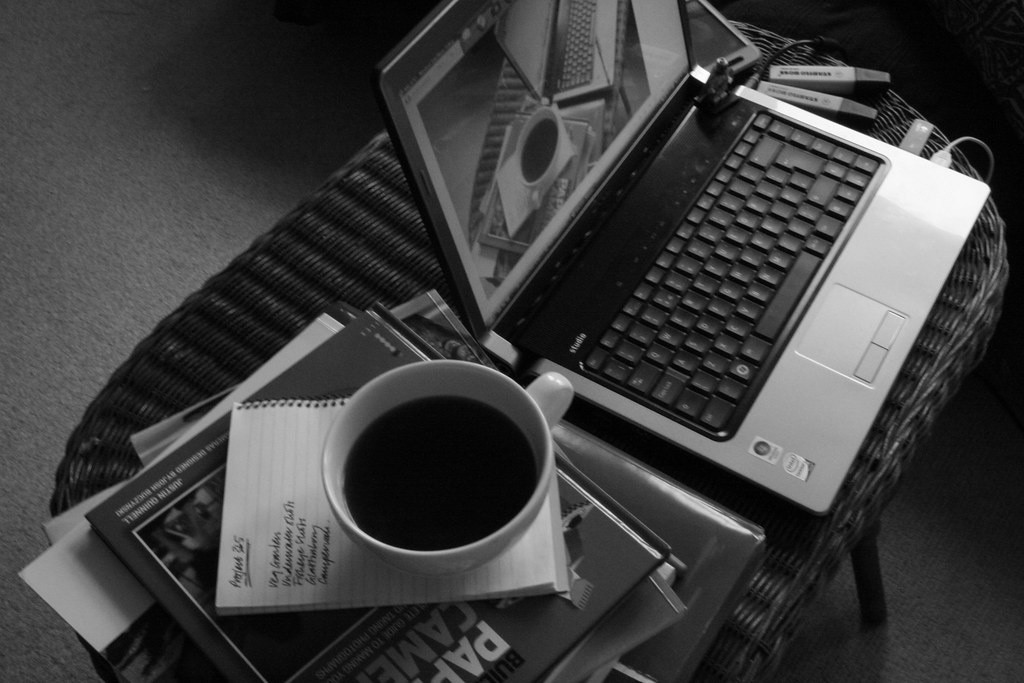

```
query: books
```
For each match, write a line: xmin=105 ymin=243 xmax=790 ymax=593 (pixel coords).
xmin=15 ymin=286 xmax=766 ymax=683
xmin=470 ymin=96 xmax=604 ymax=280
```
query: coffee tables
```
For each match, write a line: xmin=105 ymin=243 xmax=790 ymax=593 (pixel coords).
xmin=48 ymin=21 xmax=1010 ymax=683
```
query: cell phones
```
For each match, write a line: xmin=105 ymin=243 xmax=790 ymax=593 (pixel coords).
xmin=687 ymin=0 xmax=760 ymax=74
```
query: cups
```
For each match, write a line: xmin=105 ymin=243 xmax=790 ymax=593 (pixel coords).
xmin=321 ymin=359 xmax=574 ymax=580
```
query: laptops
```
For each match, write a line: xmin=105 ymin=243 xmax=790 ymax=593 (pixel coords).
xmin=495 ymin=0 xmax=618 ymax=103
xmin=372 ymin=0 xmax=991 ymax=512
